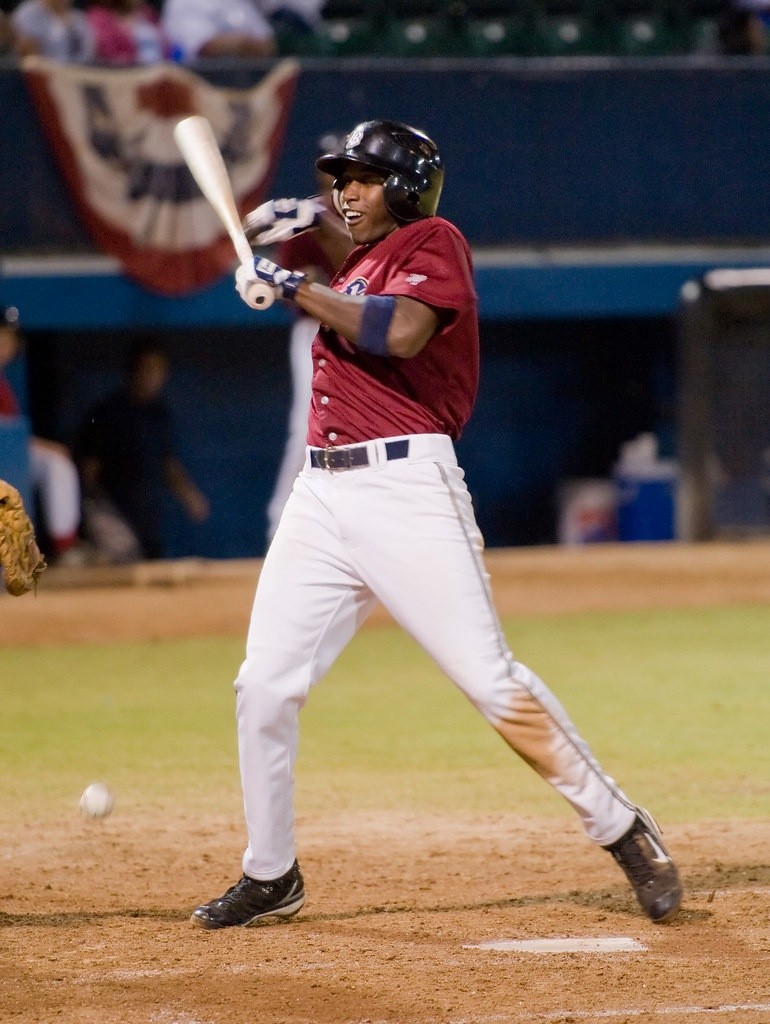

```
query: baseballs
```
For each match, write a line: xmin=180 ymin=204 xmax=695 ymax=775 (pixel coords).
xmin=75 ymin=781 xmax=120 ymax=824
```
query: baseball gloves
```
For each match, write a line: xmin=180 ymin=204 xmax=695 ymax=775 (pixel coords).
xmin=0 ymin=475 xmax=52 ymax=606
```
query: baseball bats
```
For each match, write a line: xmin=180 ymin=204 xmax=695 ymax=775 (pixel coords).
xmin=170 ymin=112 xmax=279 ymax=315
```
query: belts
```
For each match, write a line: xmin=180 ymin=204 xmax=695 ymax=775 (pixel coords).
xmin=311 ymin=439 xmax=409 ymax=474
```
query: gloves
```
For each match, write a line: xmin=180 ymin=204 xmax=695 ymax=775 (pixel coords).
xmin=241 ymin=199 xmax=314 ymax=246
xmin=235 ymin=257 xmax=303 ymax=304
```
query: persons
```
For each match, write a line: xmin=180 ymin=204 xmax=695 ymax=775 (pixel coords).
xmin=0 ymin=0 xmax=326 ymax=69
xmin=1 ymin=305 xmax=211 ymax=564
xmin=190 ymin=120 xmax=682 ymax=929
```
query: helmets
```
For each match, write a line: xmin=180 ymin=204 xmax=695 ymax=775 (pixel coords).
xmin=317 ymin=119 xmax=444 ymax=222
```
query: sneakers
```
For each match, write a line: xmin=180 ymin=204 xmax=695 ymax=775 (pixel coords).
xmin=602 ymin=808 xmax=682 ymax=923
xmin=191 ymin=858 xmax=305 ymax=929
